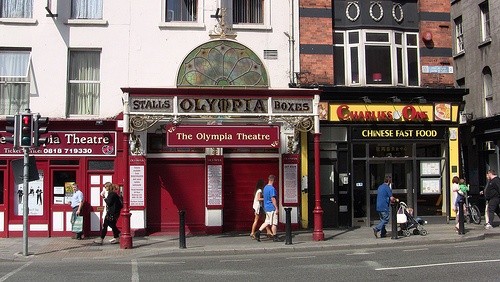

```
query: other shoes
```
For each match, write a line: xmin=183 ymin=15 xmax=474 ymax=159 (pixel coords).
xmin=372 ymin=227 xmax=378 ymax=238
xmin=484 ymin=224 xmax=494 ymax=230
xmin=381 ymin=234 xmax=390 ymax=238
xmin=271 ymin=235 xmax=283 ymax=242
xmin=455 ymin=225 xmax=460 ymax=234
xmin=252 ymin=231 xmax=261 ymax=242
xmin=109 ymin=238 xmax=120 ymax=244
xmin=72 ymin=236 xmax=81 ymax=240
xmin=94 ymin=239 xmax=103 ymax=245
xmin=250 ymin=233 xmax=256 ymax=239
xmin=267 ymin=231 xmax=273 ymax=237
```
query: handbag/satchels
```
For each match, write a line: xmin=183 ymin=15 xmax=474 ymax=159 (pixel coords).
xmin=397 ymin=206 xmax=408 ymax=224
xmin=71 ymin=214 xmax=84 ymax=234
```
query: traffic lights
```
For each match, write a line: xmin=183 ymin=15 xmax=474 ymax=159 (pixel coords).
xmin=35 ymin=114 xmax=49 ymax=146
xmin=5 ymin=114 xmax=18 ymax=149
xmin=20 ymin=113 xmax=33 ymax=147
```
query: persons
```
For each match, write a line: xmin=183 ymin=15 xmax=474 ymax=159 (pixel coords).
xmin=68 ymin=183 xmax=85 ymax=240
xmin=407 ymin=207 xmax=413 ymax=217
xmin=252 ymin=175 xmax=284 ymax=242
xmin=373 ymin=177 xmax=398 ymax=238
xmin=454 ymin=179 xmax=469 ymax=212
xmin=94 ymin=182 xmax=122 ymax=245
xmin=479 ymin=169 xmax=500 ymax=229
xmin=249 ymin=179 xmax=273 ymax=238
xmin=451 ymin=176 xmax=467 ymax=234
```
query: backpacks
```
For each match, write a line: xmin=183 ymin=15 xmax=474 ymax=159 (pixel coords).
xmin=113 ymin=193 xmax=124 ymax=211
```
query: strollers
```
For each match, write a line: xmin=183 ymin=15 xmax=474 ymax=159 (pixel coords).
xmin=393 ymin=199 xmax=428 ymax=237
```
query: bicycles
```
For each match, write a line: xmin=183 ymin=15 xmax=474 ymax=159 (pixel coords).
xmin=465 ymin=195 xmax=482 ymax=225
